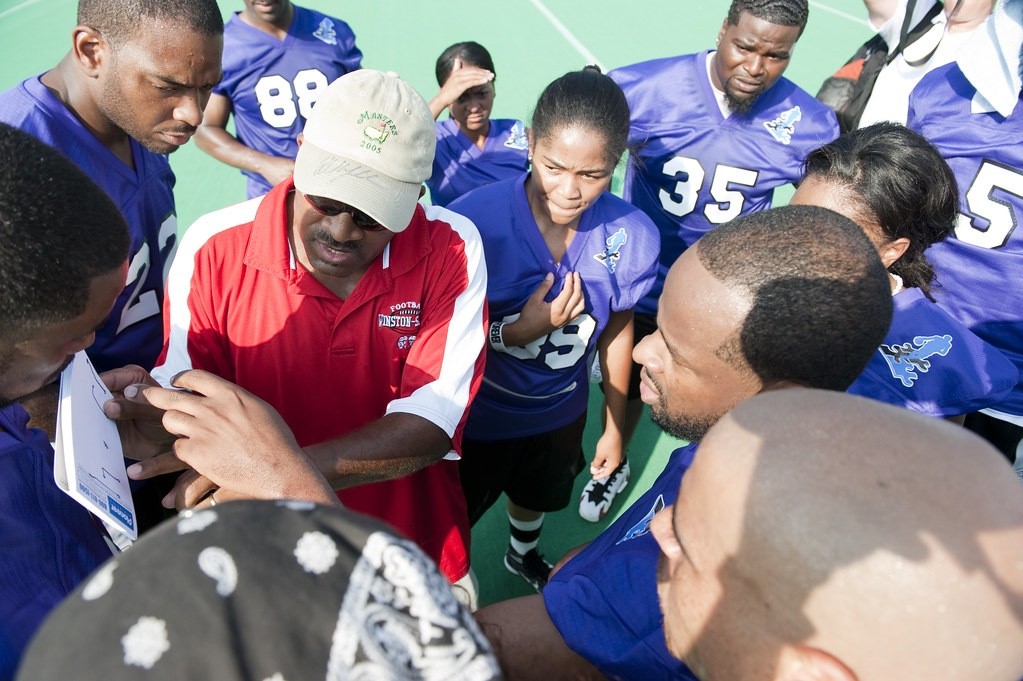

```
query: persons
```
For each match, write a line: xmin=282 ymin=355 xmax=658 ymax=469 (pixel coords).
xmin=117 ymin=200 xmax=905 ymax=681
xmin=424 ymin=42 xmax=530 ymax=209
xmin=785 ymin=119 xmax=961 ymax=297
xmin=95 ymin=67 xmax=489 ymax=610
xmin=863 ymin=0 xmax=1023 ymax=471
xmin=195 ymin=1 xmax=361 ymax=186
xmin=577 ymin=1 xmax=839 ymax=521
xmin=650 ymin=382 xmax=1023 ymax=677
xmin=0 ymin=1 xmax=223 ymax=375
xmin=0 ymin=118 xmax=132 ymax=677
xmin=21 ymin=500 xmax=502 ymax=681
xmin=444 ymin=63 xmax=659 ymax=598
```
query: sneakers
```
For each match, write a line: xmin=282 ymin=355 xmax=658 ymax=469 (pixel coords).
xmin=503 ymin=542 xmax=554 ymax=595
xmin=578 ymin=453 xmax=638 ymax=523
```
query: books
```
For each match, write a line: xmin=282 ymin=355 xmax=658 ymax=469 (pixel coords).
xmin=53 ymin=349 xmax=137 ymax=540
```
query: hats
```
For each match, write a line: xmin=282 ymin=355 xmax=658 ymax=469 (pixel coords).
xmin=292 ymin=68 xmax=436 ymax=233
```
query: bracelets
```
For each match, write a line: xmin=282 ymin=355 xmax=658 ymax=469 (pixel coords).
xmin=489 ymin=319 xmax=514 ymax=353
